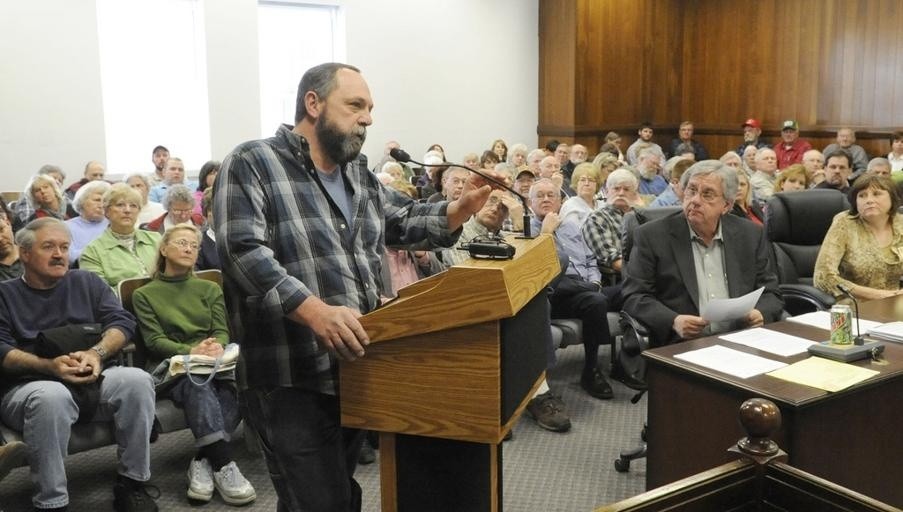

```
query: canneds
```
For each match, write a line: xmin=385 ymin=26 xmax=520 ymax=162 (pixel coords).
xmin=829 ymin=303 xmax=853 ymax=346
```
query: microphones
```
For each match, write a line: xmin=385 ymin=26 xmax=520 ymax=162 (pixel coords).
xmin=386 ymin=146 xmax=541 ymax=240
xmin=806 ymin=283 xmax=885 ymax=365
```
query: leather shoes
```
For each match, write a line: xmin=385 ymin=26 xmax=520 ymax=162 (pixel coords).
xmin=582 ymin=363 xmax=613 ymax=399
xmin=608 ymin=360 xmax=648 ymax=389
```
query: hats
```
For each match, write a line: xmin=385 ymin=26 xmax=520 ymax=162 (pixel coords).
xmin=741 ymin=119 xmax=761 ymax=128
xmin=780 ymin=120 xmax=799 ymax=131
xmin=510 ymin=165 xmax=536 ymax=184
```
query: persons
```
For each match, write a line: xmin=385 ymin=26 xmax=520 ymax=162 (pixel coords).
xmin=0 ymin=217 xmax=164 ymax=511
xmin=621 ymin=159 xmax=785 ymax=348
xmin=1 ymin=145 xmax=224 ymax=299
xmin=131 ymin=222 xmax=258 ymax=506
xmin=374 ymin=121 xmax=710 ymax=433
xmin=209 ymin=61 xmax=513 ymax=511
xmin=721 ymin=117 xmax=903 ymax=308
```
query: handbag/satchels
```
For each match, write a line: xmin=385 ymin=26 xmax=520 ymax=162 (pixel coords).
xmin=35 ymin=323 xmax=118 ymax=370
xmin=552 ymin=274 xmax=594 ymax=296
xmin=168 ymin=343 xmax=239 ymax=387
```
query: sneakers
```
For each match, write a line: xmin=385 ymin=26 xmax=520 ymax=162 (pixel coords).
xmin=213 ymin=460 xmax=256 ymax=505
xmin=358 ymin=430 xmax=375 ymax=464
xmin=526 ymin=387 xmax=570 ymax=431
xmin=186 ymin=455 xmax=215 ymax=501
xmin=112 ymin=472 xmax=161 ymax=512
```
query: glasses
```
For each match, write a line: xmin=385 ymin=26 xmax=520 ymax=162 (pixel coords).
xmin=168 ymin=205 xmax=192 ymax=215
xmin=166 ymin=239 xmax=199 ymax=251
xmin=684 ymin=186 xmax=723 ymax=204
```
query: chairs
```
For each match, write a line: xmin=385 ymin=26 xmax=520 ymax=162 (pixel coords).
xmin=0 ymin=191 xmax=23 ymax=211
xmin=764 ymin=189 xmax=852 ymax=310
xmin=616 ymin=207 xmax=795 ymax=475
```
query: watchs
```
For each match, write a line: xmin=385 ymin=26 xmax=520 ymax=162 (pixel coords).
xmin=93 ymin=345 xmax=112 ymax=368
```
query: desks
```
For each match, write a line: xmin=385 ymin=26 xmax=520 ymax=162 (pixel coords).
xmin=642 ymin=295 xmax=903 ymax=512
